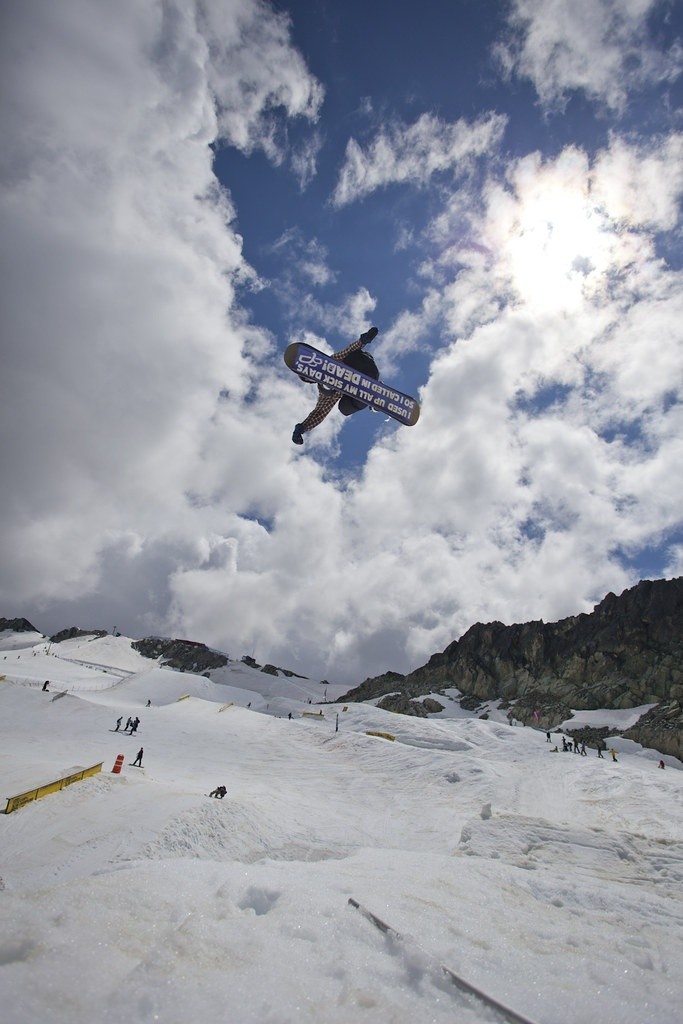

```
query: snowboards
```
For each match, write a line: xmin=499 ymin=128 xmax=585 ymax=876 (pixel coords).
xmin=284 ymin=341 xmax=419 ymax=426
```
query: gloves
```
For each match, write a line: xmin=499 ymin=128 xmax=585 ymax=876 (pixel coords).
xmin=292 ymin=423 xmax=304 ymax=445
xmin=360 ymin=327 xmax=378 ymax=344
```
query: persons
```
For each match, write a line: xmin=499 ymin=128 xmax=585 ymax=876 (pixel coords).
xmin=607 ymin=749 xmax=618 ymax=762
xmin=562 ymin=737 xmax=567 ymax=750
xmin=288 ymin=713 xmax=291 ymax=720
xmin=115 ymin=716 xmax=123 ymax=732
xmin=546 ymin=731 xmax=551 ymax=742
xmin=145 ymin=700 xmax=151 ymax=707
xmin=124 ymin=717 xmax=132 ymax=731
xmin=132 ymin=747 xmax=143 ymax=767
xmin=41 ymin=680 xmax=48 ymax=691
xmin=597 ymin=746 xmax=604 ymax=758
xmin=572 ymin=739 xmax=579 ymax=753
xmin=290 ymin=327 xmax=382 ymax=445
xmin=567 ymin=742 xmax=572 ymax=751
xmin=658 ymin=759 xmax=664 ymax=769
xmin=129 ymin=717 xmax=138 ymax=735
xmin=580 ymin=741 xmax=587 ymax=755
xmin=209 ymin=786 xmax=226 ymax=799
